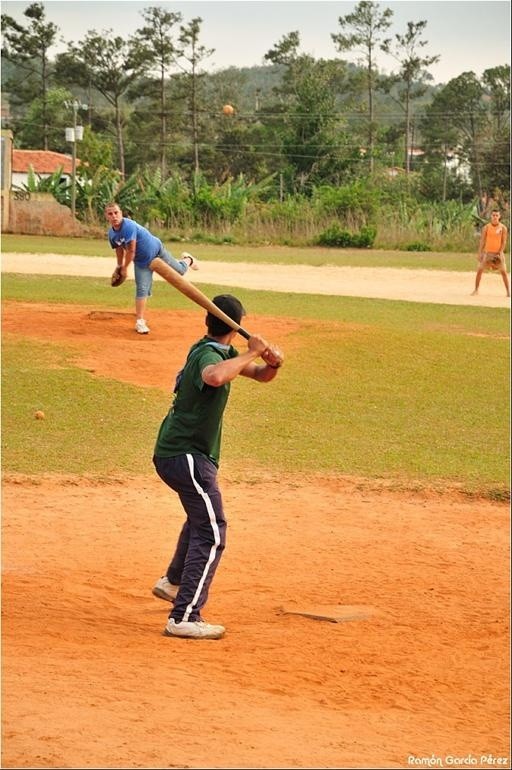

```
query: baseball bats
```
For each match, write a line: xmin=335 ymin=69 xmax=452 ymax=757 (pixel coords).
xmin=149 ymin=257 xmax=280 ymax=367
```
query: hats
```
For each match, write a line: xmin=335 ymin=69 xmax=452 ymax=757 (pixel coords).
xmin=210 ymin=294 xmax=246 ymax=328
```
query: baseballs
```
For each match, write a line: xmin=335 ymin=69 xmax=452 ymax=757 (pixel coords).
xmin=34 ymin=411 xmax=45 ymax=419
xmin=224 ymin=105 xmax=234 ymax=115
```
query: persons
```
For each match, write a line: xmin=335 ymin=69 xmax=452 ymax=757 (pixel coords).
xmin=153 ymin=295 xmax=283 ymax=638
xmin=104 ymin=201 xmax=200 ymax=335
xmin=471 ymin=210 xmax=511 ymax=297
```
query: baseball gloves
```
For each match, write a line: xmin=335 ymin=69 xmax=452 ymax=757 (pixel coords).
xmin=111 ymin=266 xmax=126 ymax=287
xmin=487 ymin=255 xmax=502 ymax=269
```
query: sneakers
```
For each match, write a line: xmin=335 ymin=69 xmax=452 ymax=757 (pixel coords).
xmin=135 ymin=319 xmax=151 ymax=334
xmin=152 ymin=575 xmax=181 ymax=603
xmin=164 ymin=617 xmax=226 ymax=639
xmin=181 ymin=252 xmax=202 ymax=271
